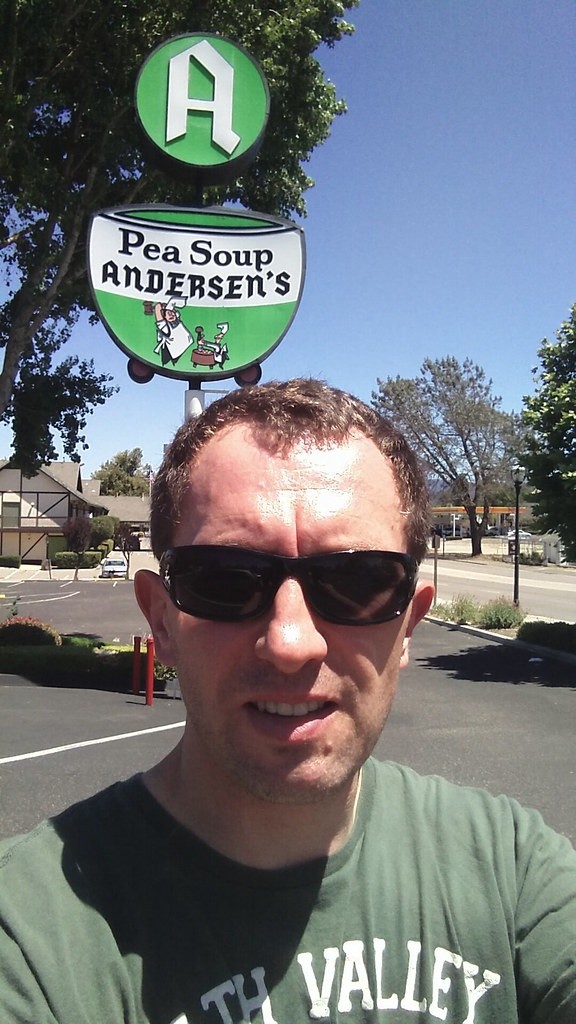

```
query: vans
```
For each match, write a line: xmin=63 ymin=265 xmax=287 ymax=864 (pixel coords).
xmin=438 ymin=523 xmax=467 ymax=537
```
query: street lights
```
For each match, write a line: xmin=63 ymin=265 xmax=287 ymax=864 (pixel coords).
xmin=451 ymin=514 xmax=455 ymax=537
xmin=510 ymin=514 xmax=515 ymax=529
xmin=511 ymin=460 xmax=526 ymax=607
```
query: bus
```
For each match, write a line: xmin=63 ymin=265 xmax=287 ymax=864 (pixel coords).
xmin=129 ymin=525 xmax=151 ymax=537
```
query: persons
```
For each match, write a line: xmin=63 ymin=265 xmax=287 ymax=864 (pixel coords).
xmin=0 ymin=380 xmax=576 ymax=1024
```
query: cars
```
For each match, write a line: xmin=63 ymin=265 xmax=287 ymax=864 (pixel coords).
xmin=485 ymin=527 xmax=498 ymax=536
xmin=101 ymin=557 xmax=127 ymax=577
xmin=507 ymin=530 xmax=532 ymax=536
xmin=123 ymin=537 xmax=142 ymax=550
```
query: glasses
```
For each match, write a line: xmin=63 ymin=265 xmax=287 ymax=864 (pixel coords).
xmin=159 ymin=545 xmax=419 ymax=626
